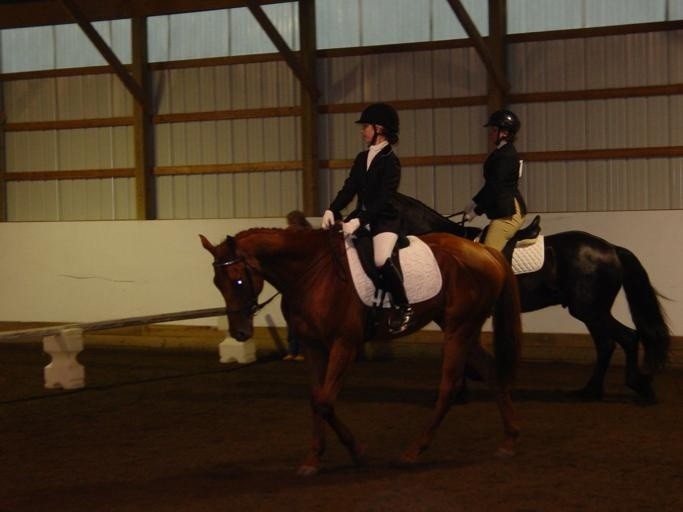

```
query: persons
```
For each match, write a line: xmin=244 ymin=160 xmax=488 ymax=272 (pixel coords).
xmin=321 ymin=101 xmax=415 ymax=338
xmin=465 ymin=109 xmax=527 ymax=267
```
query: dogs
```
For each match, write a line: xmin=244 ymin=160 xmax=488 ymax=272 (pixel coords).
xmin=198 ymin=226 xmax=527 ymax=474
xmin=390 ymin=189 xmax=673 ymax=404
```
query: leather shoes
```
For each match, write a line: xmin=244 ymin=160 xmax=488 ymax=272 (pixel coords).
xmin=343 ymin=217 xmax=360 ymax=235
xmin=322 ymin=210 xmax=335 ymax=230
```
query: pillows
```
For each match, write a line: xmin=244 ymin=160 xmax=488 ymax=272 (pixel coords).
xmin=483 ymin=110 xmax=520 ymax=131
xmin=356 ymin=103 xmax=399 ymax=127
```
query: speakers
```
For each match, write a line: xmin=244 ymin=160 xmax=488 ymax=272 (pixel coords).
xmin=377 ymin=258 xmax=413 ymax=335
